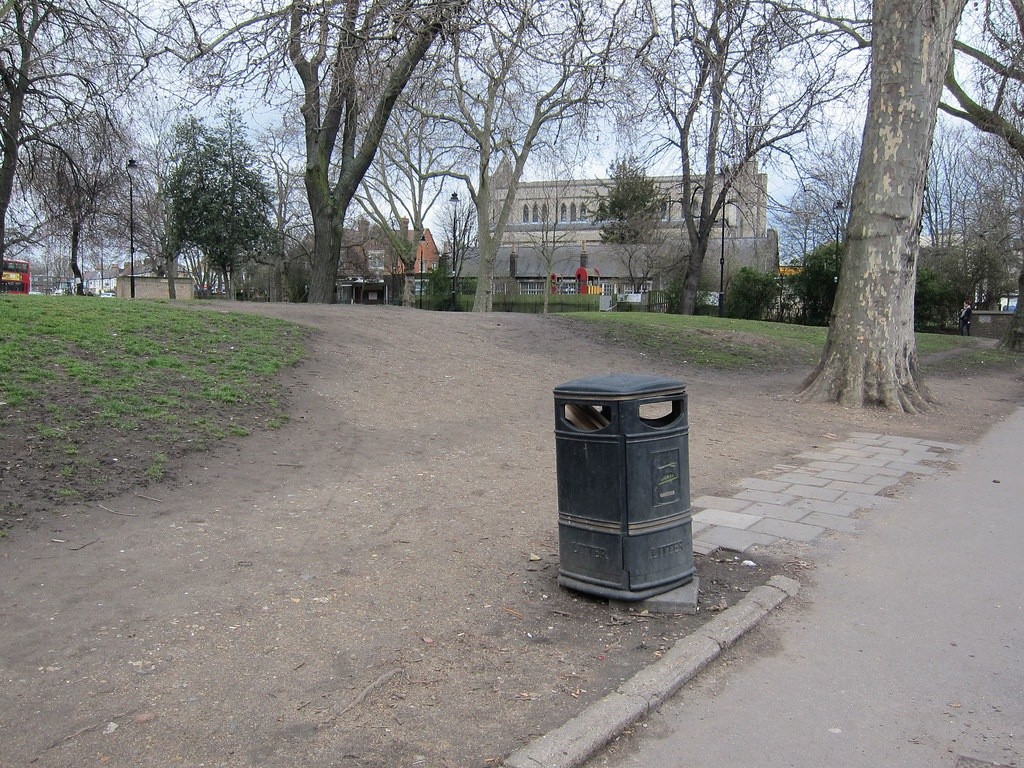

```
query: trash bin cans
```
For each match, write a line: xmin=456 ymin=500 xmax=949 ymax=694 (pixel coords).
xmin=553 ymin=375 xmax=696 ymax=603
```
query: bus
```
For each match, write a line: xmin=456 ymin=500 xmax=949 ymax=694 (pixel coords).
xmin=0 ymin=258 xmax=30 ymax=295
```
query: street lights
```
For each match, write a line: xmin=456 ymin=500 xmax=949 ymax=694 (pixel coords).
xmin=449 ymin=191 xmax=459 ymax=308
xmin=125 ymin=155 xmax=139 ymax=299
xmin=833 ymin=199 xmax=845 ymax=281
xmin=418 ymin=236 xmax=427 ymax=310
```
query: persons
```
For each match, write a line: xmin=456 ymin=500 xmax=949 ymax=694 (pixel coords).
xmin=958 ymin=300 xmax=973 ymax=336
